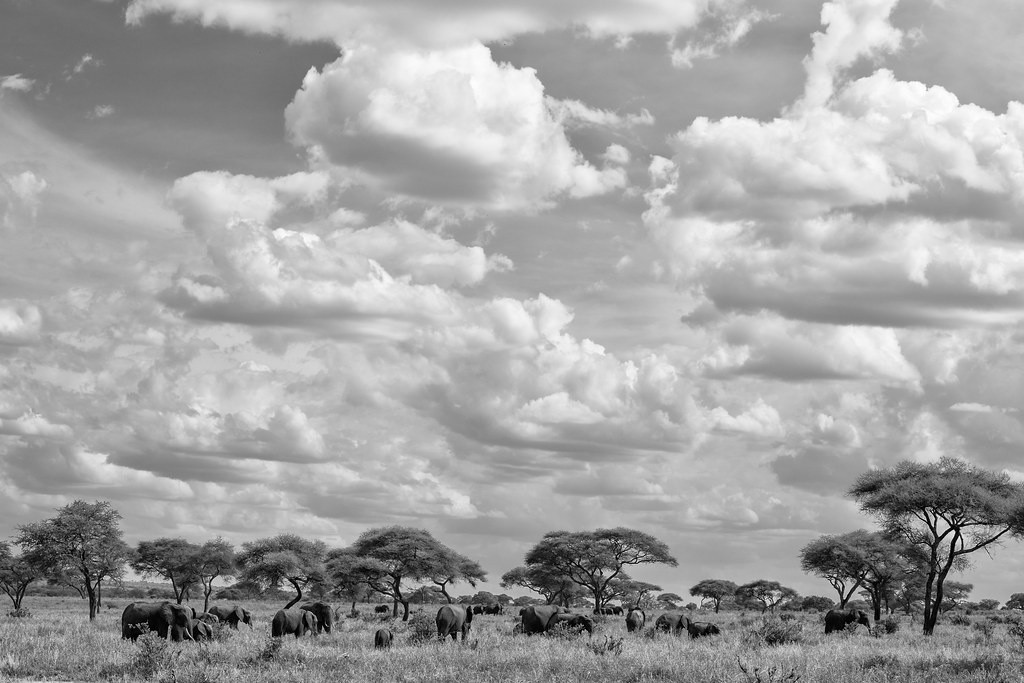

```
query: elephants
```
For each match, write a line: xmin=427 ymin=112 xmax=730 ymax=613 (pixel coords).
xmin=825 ymin=608 xmax=873 ymax=636
xmin=625 ymin=607 xmax=645 ymax=632
xmin=473 ymin=603 xmax=500 ymax=616
xmin=435 ymin=605 xmax=473 ymax=642
xmin=375 ymin=629 xmax=394 ymax=652
xmin=593 ymin=605 xmax=624 ymax=617
xmin=121 ymin=601 xmax=254 ymax=644
xmin=655 ymin=613 xmax=721 ymax=639
xmin=375 ymin=605 xmax=389 ymax=613
xmin=519 ymin=605 xmax=593 ymax=638
xmin=272 ymin=602 xmax=336 ymax=639
